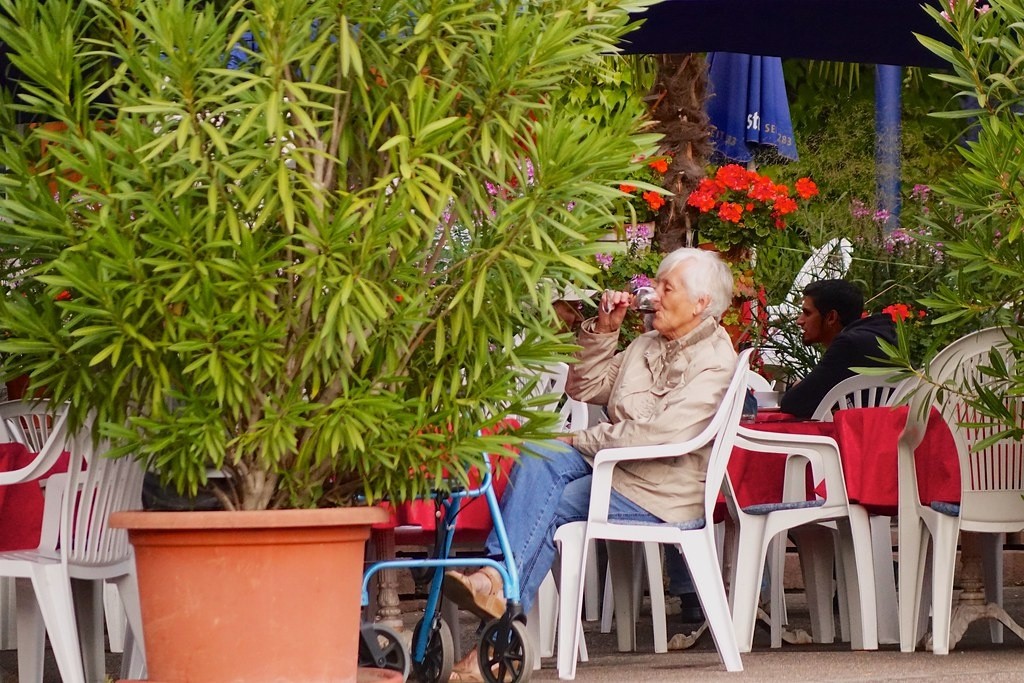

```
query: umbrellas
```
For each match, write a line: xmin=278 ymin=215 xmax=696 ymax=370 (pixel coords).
xmin=703 ymin=52 xmax=798 ymax=345
xmin=111 ymin=7 xmax=425 ymax=97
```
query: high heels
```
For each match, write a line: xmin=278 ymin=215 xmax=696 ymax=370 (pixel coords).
xmin=667 ymin=619 xmax=708 ymax=650
xmin=756 ymin=608 xmax=812 ymax=645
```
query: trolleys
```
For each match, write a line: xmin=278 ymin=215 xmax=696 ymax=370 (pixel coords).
xmin=351 ymin=425 xmax=534 ymax=683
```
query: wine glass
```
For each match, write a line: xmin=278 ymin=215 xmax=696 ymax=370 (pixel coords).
xmin=602 ymin=287 xmax=662 ymax=315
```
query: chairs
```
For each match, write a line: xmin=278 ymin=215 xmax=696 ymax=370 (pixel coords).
xmin=0 ymin=238 xmax=1024 ymax=683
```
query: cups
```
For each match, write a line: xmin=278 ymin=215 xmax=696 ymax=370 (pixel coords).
xmin=753 ymin=391 xmax=780 ymax=406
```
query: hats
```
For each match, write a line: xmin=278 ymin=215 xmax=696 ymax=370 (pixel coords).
xmin=522 ymin=276 xmax=597 ymax=313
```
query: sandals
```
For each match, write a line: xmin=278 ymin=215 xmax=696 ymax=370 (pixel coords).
xmin=444 ymin=641 xmax=520 ymax=683
xmin=443 ymin=566 xmax=507 ymax=621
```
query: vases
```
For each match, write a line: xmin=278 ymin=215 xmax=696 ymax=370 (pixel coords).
xmin=594 ymin=221 xmax=656 ymax=264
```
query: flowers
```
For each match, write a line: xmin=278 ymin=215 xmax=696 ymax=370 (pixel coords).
xmin=403 ymin=140 xmax=819 ymax=235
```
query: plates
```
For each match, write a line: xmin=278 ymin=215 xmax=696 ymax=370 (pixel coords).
xmin=756 ymin=407 xmax=782 ymax=411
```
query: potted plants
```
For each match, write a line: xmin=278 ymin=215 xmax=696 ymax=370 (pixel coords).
xmin=0 ymin=0 xmax=144 ymax=214
xmin=0 ymin=0 xmax=671 ymax=683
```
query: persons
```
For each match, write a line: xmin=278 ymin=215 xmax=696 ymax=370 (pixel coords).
xmin=501 ymin=275 xmax=597 ymax=363
xmin=664 ymin=385 xmax=771 ymax=622
xmin=778 ymin=280 xmax=899 ymax=617
xmin=138 ymin=397 xmax=243 ymax=513
xmin=440 ymin=247 xmax=740 ymax=683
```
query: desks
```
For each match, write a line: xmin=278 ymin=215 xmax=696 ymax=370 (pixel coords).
xmin=815 ymin=406 xmax=1024 ymax=646
xmin=715 ymin=422 xmax=836 ymax=641
xmin=360 ymin=432 xmax=523 ymax=660
